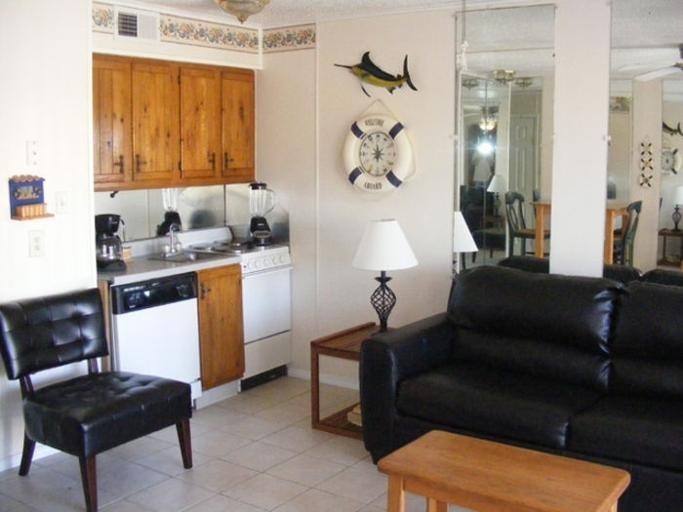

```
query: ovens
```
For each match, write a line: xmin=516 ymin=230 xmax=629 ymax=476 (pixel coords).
xmin=241 ymin=264 xmax=292 ymax=391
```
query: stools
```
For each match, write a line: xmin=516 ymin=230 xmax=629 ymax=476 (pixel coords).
xmin=657 ymin=228 xmax=683 ymax=264
xmin=377 ymin=430 xmax=632 ymax=512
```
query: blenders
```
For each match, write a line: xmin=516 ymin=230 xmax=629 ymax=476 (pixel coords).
xmin=162 ymin=187 xmax=187 ymax=231
xmin=248 ymin=181 xmax=277 ymax=237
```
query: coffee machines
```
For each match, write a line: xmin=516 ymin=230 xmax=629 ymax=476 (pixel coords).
xmin=95 ymin=214 xmax=126 ymax=273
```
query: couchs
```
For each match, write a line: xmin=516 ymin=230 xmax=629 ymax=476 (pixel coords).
xmin=500 ymin=256 xmax=683 ymax=287
xmin=359 ymin=266 xmax=682 ymax=512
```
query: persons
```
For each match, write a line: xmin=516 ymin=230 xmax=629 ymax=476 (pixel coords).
xmin=471 ymin=150 xmax=494 ymax=191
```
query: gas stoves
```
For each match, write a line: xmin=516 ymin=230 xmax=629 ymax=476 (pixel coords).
xmin=183 ymin=226 xmax=290 ymax=275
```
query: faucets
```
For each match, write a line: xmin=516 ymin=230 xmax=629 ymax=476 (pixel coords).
xmin=169 ymin=222 xmax=182 ymax=255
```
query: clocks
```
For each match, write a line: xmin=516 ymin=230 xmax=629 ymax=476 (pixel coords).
xmin=342 ymin=115 xmax=413 ymax=194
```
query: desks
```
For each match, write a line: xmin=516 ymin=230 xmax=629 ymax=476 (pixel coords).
xmin=529 ymin=200 xmax=631 ymax=264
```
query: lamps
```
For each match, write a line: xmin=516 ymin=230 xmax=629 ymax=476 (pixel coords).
xmin=462 ymin=68 xmax=534 ymax=132
xmin=672 ymin=185 xmax=683 ymax=232
xmin=351 ymin=219 xmax=419 ymax=337
xmin=661 ymin=137 xmax=681 ymax=175
xmin=486 ymin=173 xmax=507 ymax=217
xmin=454 ymin=211 xmax=479 ymax=274
xmin=216 ymin=0 xmax=271 ymax=25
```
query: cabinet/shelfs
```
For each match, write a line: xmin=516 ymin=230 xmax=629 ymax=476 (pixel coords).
xmin=198 ymin=264 xmax=245 ymax=391
xmin=181 ymin=62 xmax=255 ymax=187
xmin=92 ymin=53 xmax=180 ymax=192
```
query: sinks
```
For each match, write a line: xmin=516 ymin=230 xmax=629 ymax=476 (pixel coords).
xmin=146 ymin=248 xmax=238 ymax=263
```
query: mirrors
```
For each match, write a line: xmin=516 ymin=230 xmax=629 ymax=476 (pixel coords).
xmin=455 ymin=2 xmax=556 ymax=274
xmin=604 ymin=0 xmax=683 ymax=267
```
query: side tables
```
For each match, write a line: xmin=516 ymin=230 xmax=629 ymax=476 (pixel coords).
xmin=310 ymin=321 xmax=398 ymax=440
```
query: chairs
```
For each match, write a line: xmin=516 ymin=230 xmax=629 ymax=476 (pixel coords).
xmin=0 ymin=288 xmax=193 ymax=512
xmin=531 ymin=188 xmax=541 ymax=214
xmin=613 ymin=199 xmax=642 ymax=265
xmin=503 ymin=192 xmax=551 ymax=256
